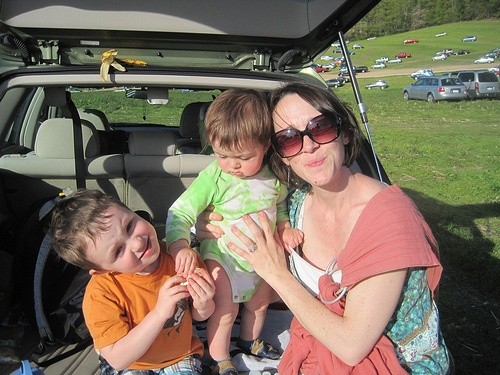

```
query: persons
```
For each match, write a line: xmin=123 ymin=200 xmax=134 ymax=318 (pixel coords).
xmin=50 ymin=189 xmax=216 ymax=375
xmin=195 ymin=83 xmax=456 ymax=375
xmin=169 ymin=88 xmax=304 ymax=375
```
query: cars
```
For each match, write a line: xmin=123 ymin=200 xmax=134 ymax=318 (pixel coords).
xmin=495 ymin=71 xmax=500 ymax=76
xmin=463 ymin=36 xmax=477 ymax=42
xmin=403 ymin=38 xmax=418 ymax=44
xmin=0 ymin=0 xmax=391 ymax=374
xmin=388 ymin=59 xmax=402 ymax=63
xmin=365 ymin=80 xmax=389 ymax=90
xmin=315 ymin=40 xmax=368 ymax=88
xmin=371 ymin=64 xmax=385 ymax=69
xmin=434 ymin=31 xmax=447 ymax=38
xmin=374 ymin=57 xmax=388 ymax=64
xmin=395 ymin=52 xmax=411 ymax=59
xmin=444 ymin=70 xmax=500 ymax=98
xmin=403 ymin=75 xmax=468 ymax=104
xmin=453 ymin=50 xmax=470 ymax=56
xmin=474 ymin=48 xmax=500 ymax=64
xmin=487 ymin=65 xmax=500 ymax=73
xmin=431 ymin=49 xmax=453 ymax=61
xmin=411 ymin=69 xmax=434 ymax=80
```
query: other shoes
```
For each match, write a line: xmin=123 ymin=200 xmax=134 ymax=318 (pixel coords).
xmin=239 ymin=337 xmax=280 ymax=360
xmin=218 ymin=358 xmax=238 ymax=375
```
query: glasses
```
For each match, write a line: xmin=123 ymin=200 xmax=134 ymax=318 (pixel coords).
xmin=273 ymin=112 xmax=342 ymax=158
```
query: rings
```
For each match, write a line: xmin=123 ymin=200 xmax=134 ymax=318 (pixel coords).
xmin=248 ymin=244 xmax=257 ymax=253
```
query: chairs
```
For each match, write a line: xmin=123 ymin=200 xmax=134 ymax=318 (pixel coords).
xmin=0 ymin=100 xmax=214 ymax=238
